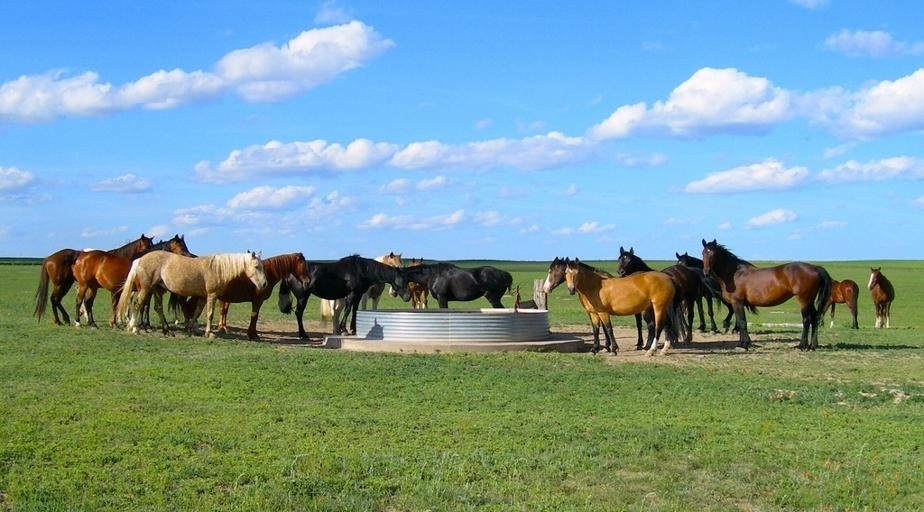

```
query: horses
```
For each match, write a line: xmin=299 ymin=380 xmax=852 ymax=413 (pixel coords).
xmin=33 ymin=234 xmax=155 ymax=326
xmin=168 ymin=253 xmax=311 ymax=340
xmin=702 ymin=238 xmax=832 ymax=353
xmin=321 ymin=253 xmax=404 ymax=334
xmin=818 ymin=279 xmax=860 ymax=329
xmin=676 ymin=252 xmax=759 ymax=336
xmin=408 ymin=258 xmax=429 ymax=310
xmin=566 ymin=257 xmax=688 ymax=357
xmin=866 ymin=267 xmax=905 ymax=328
xmin=390 ymin=263 xmax=513 ymax=308
xmin=279 ymin=255 xmax=415 ymax=334
xmin=542 ymin=258 xmax=655 ymax=355
xmin=62 ymin=234 xmax=193 ymax=333
xmin=616 ymin=247 xmax=721 ymax=350
